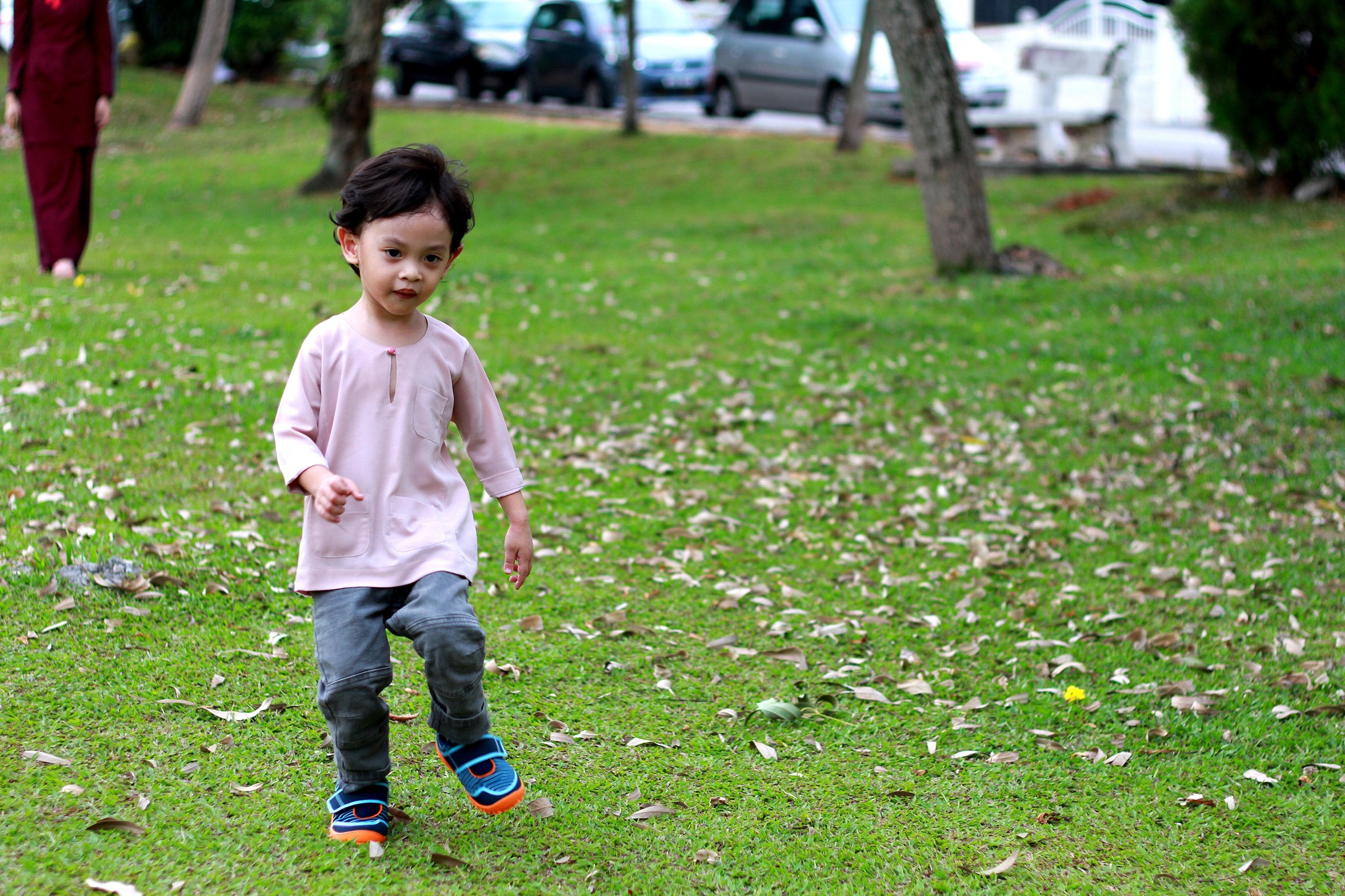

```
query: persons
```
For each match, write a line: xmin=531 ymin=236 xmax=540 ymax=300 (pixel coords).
xmin=272 ymin=144 xmax=535 ymax=847
xmin=4 ymin=0 xmax=116 ymax=277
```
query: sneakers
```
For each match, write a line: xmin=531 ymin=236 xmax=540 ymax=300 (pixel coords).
xmin=435 ymin=729 xmax=526 ymax=814
xmin=326 ymin=777 xmax=391 ymax=846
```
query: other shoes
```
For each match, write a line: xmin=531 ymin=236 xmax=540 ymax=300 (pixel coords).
xmin=53 ymin=259 xmax=73 ymax=277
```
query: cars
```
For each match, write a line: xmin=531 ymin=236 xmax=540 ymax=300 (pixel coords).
xmin=704 ymin=0 xmax=1015 ymax=127
xmin=373 ymin=0 xmax=722 ymax=115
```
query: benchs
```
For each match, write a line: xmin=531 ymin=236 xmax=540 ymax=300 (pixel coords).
xmin=966 ymin=49 xmax=1139 ymax=170
